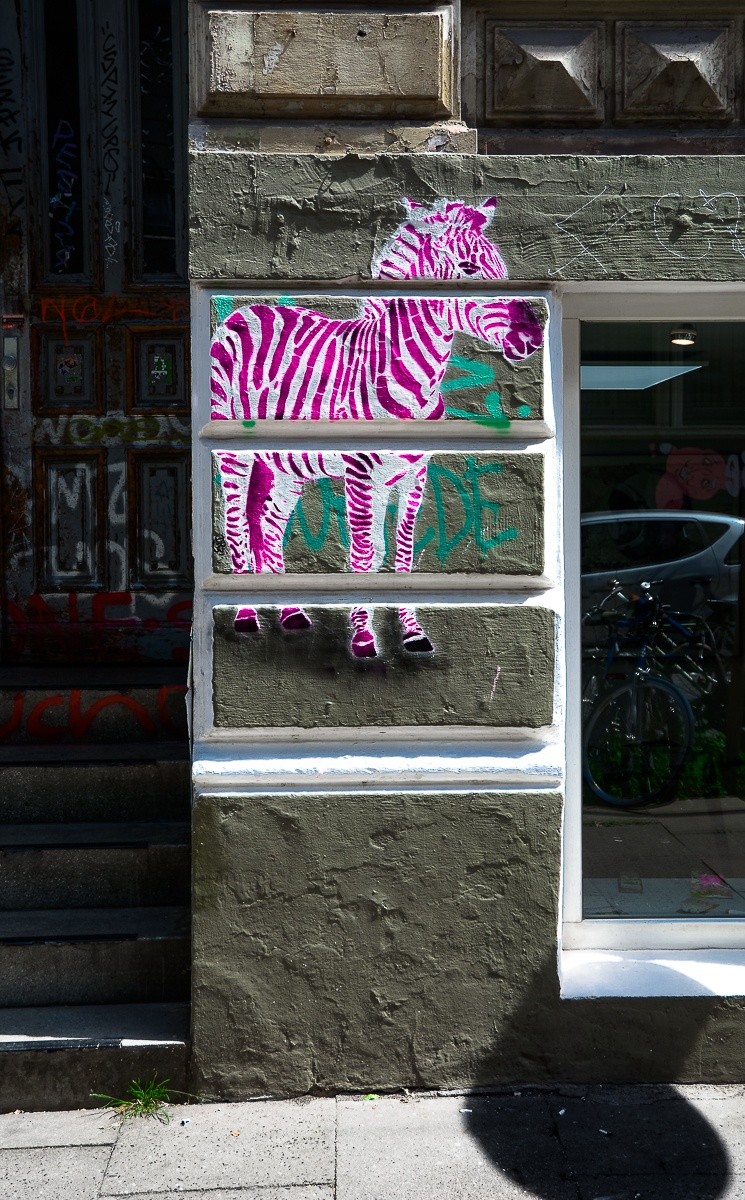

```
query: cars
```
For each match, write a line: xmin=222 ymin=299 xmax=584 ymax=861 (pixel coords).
xmin=580 ymin=506 xmax=745 ymax=689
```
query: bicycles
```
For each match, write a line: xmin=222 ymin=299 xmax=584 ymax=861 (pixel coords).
xmin=579 ymin=577 xmax=745 ymax=808
